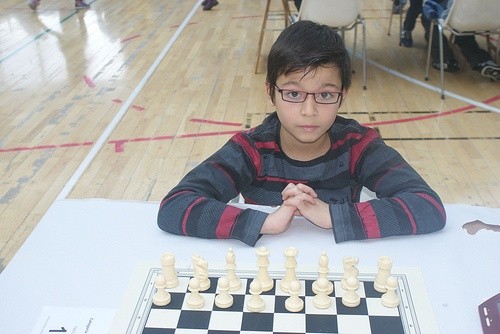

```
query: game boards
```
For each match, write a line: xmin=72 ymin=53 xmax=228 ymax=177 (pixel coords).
xmin=125 ymin=266 xmax=422 ymax=334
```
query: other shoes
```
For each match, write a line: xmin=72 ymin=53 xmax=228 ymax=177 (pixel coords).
xmin=202 ymin=0 xmax=218 ymax=10
xmin=400 ymin=29 xmax=413 ymax=47
xmin=431 ymin=59 xmax=460 ymax=74
xmin=75 ymin=2 xmax=90 ymax=9
xmin=476 ymin=59 xmax=500 ymax=81
xmin=393 ymin=5 xmax=403 ymax=14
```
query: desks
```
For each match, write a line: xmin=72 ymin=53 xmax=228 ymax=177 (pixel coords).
xmin=0 ymin=199 xmax=500 ymax=334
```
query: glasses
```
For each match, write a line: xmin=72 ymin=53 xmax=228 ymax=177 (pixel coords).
xmin=269 ymin=76 xmax=342 ymax=104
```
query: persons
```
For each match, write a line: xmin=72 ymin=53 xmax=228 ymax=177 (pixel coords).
xmin=158 ymin=20 xmax=446 ymax=243
xmin=201 ymin=0 xmax=219 ymax=10
xmin=28 ymin=0 xmax=90 ymax=9
xmin=393 ymin=0 xmax=500 ymax=80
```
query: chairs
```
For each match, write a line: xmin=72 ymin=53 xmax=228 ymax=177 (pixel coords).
xmin=287 ymin=0 xmax=500 ymax=100
